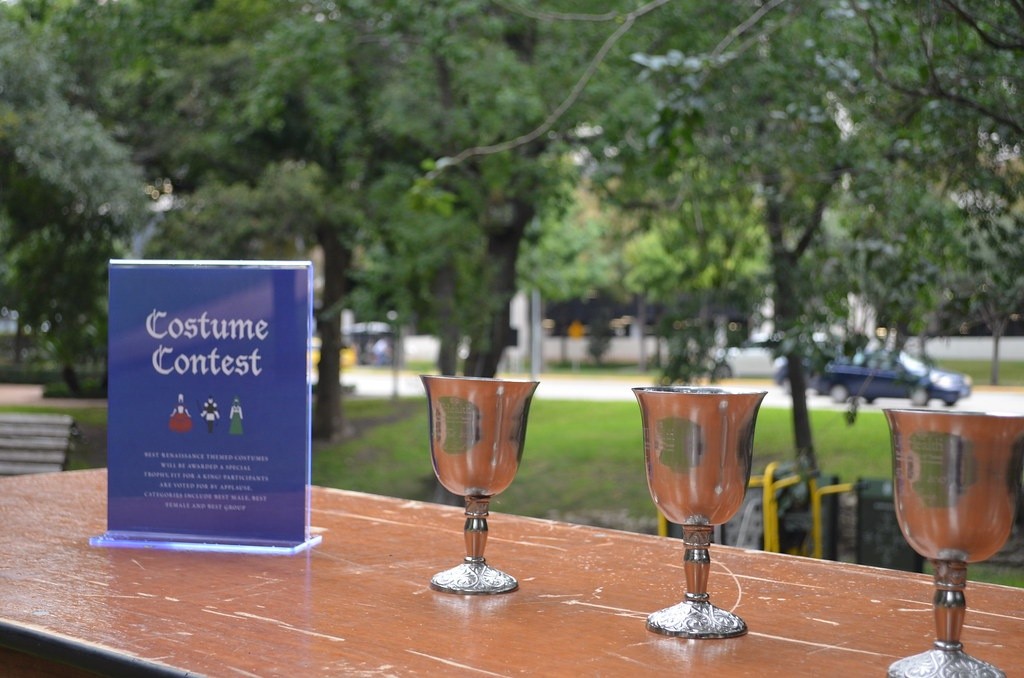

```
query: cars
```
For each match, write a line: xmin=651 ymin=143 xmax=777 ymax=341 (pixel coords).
xmin=772 ymin=330 xmax=974 ymax=405
xmin=312 ymin=334 xmax=357 ymax=371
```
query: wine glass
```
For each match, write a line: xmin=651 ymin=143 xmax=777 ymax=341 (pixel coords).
xmin=419 ymin=374 xmax=540 ymax=596
xmin=631 ymin=388 xmax=768 ymax=639
xmin=881 ymin=406 xmax=1024 ymax=678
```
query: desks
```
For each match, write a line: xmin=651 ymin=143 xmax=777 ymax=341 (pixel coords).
xmin=1 ymin=468 xmax=1024 ymax=678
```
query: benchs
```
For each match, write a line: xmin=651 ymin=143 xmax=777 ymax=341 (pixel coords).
xmin=0 ymin=410 xmax=80 ymax=476
